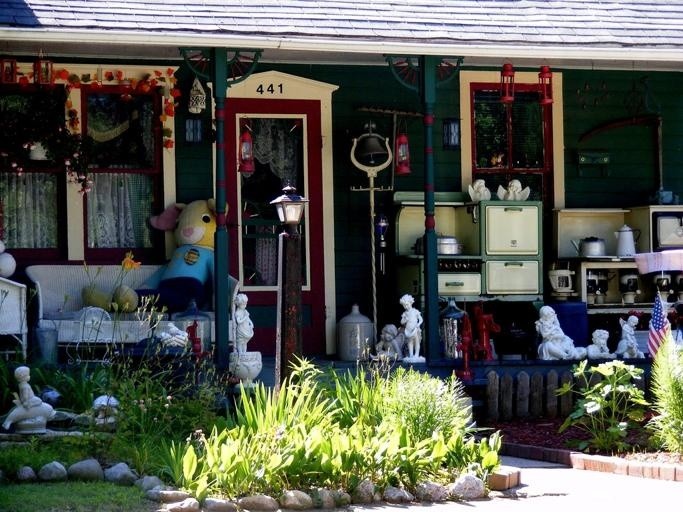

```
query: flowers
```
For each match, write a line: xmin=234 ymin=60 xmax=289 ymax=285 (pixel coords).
xmin=8 ymin=126 xmax=93 ymax=196
xmin=20 ymin=61 xmax=181 ymax=149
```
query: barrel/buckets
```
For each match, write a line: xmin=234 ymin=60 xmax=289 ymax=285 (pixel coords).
xmin=439 ymin=297 xmax=470 ymax=359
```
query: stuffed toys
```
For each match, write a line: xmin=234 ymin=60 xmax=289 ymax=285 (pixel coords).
xmin=83 ymin=199 xmax=229 ymax=314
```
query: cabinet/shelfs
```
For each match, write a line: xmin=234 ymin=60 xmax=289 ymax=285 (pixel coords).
xmin=396 ymin=201 xmax=543 ymax=302
xmin=552 ymin=206 xmax=683 ymax=313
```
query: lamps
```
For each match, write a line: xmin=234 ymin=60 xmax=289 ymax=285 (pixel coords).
xmin=270 ymin=176 xmax=309 ymax=236
xmin=238 ymin=132 xmax=254 ymax=172
xmin=395 ymin=135 xmax=412 ymax=175
xmin=538 ymin=66 xmax=553 ymax=104
xmin=501 ymin=64 xmax=514 ymax=103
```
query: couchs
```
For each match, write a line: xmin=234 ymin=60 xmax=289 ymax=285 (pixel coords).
xmin=26 ymin=264 xmax=241 ymax=365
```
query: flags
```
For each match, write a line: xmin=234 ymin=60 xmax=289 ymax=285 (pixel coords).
xmin=648 ymin=290 xmax=672 ymax=364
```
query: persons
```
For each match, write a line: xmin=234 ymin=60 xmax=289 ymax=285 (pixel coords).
xmin=14 ymin=366 xmax=42 ymax=411
xmin=370 ymin=325 xmax=404 ymax=362
xmin=534 ymin=306 xmax=588 ymax=360
xmin=468 ymin=179 xmax=491 ymax=201
xmin=497 ymin=179 xmax=530 ymax=202
xmin=399 ymin=294 xmax=425 ymax=363
xmin=587 ymin=330 xmax=616 ymax=360
xmin=617 ymin=315 xmax=645 ymax=360
xmin=234 ymin=294 xmax=254 ymax=354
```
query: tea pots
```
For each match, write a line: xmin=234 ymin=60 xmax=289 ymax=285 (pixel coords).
xmin=613 ymin=224 xmax=641 ymax=256
xmin=570 ymin=236 xmax=607 ymax=257
